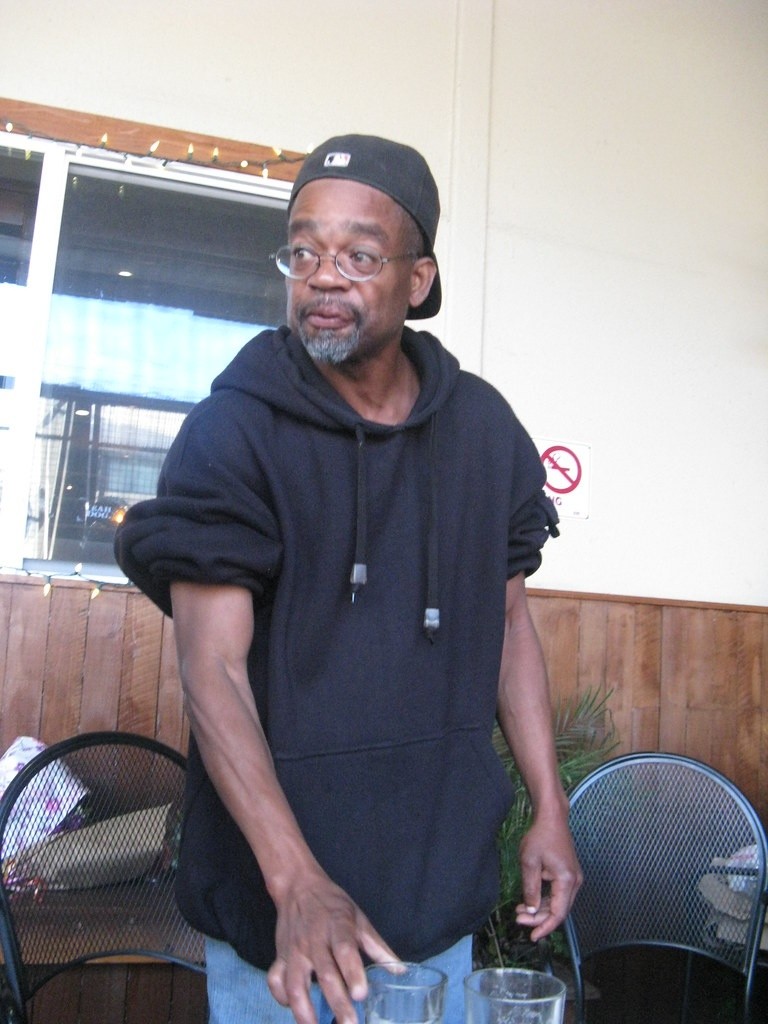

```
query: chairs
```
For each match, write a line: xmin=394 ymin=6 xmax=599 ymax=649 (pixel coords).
xmin=0 ymin=731 xmax=207 ymax=1024
xmin=562 ymin=750 xmax=768 ymax=1024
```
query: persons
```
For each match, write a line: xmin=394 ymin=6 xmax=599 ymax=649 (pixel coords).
xmin=112 ymin=134 xmax=587 ymax=1022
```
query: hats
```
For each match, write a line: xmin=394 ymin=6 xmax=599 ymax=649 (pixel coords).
xmin=288 ymin=134 xmax=442 ymax=321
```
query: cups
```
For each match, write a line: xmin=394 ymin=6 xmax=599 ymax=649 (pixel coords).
xmin=463 ymin=968 xmax=566 ymax=1023
xmin=363 ymin=963 xmax=448 ymax=1023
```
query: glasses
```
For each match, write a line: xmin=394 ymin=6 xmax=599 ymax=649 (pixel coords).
xmin=269 ymin=243 xmax=418 ymax=282
